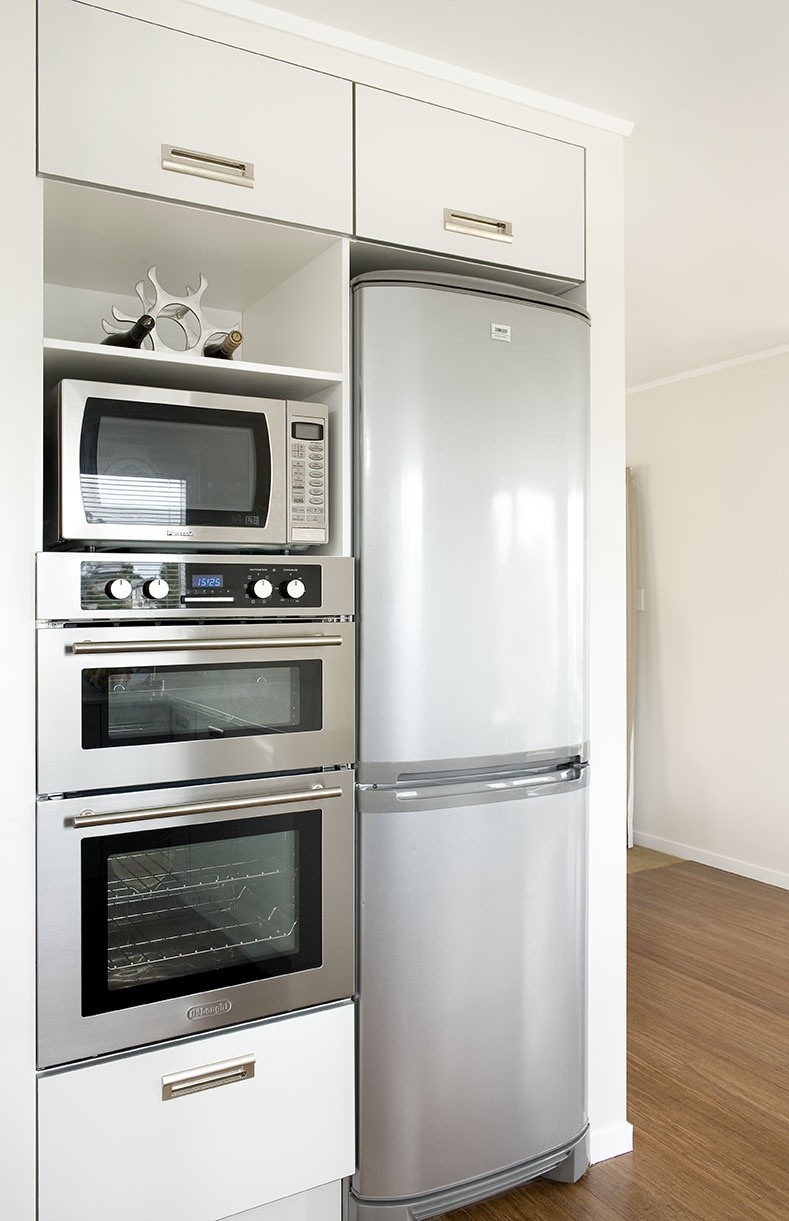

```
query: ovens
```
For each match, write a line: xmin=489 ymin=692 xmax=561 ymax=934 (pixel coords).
xmin=33 ymin=552 xmax=358 ymax=1221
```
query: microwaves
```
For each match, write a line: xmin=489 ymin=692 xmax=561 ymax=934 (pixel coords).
xmin=45 ymin=375 xmax=333 ymax=558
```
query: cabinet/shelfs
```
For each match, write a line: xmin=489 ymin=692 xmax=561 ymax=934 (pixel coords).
xmin=36 ymin=0 xmax=586 ymax=1221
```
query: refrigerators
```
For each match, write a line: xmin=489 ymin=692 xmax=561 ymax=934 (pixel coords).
xmin=343 ymin=267 xmax=596 ymax=1218
